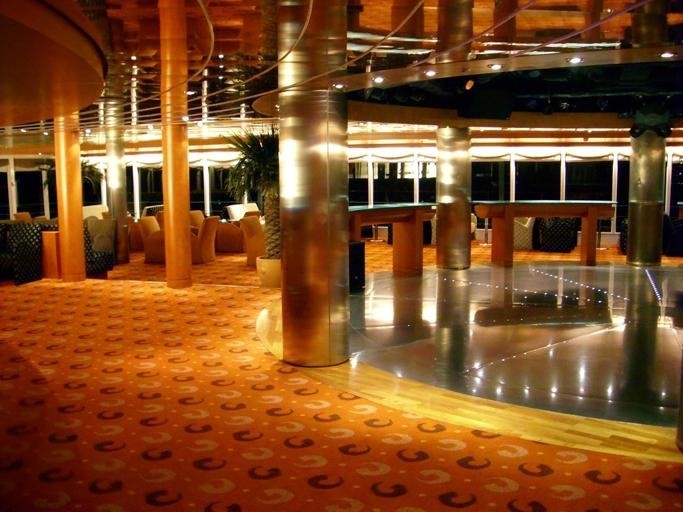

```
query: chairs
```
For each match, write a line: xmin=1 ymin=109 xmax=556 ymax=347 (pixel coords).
xmin=513 ymin=217 xmax=536 ymax=249
xmin=532 ymin=216 xmax=580 ymax=252
xmin=431 ymin=206 xmax=477 ymax=246
xmin=0 ymin=202 xmax=267 ymax=284
xmin=620 ymin=214 xmax=683 ymax=257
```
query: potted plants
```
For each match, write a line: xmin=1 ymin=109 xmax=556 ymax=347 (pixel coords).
xmin=219 ymin=119 xmax=283 ymax=287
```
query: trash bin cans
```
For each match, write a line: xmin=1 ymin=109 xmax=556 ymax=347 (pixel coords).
xmin=349 ymin=240 xmax=365 ymax=292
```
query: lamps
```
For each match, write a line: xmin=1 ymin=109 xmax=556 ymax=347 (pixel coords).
xmin=513 ymin=91 xmax=683 ymax=137
